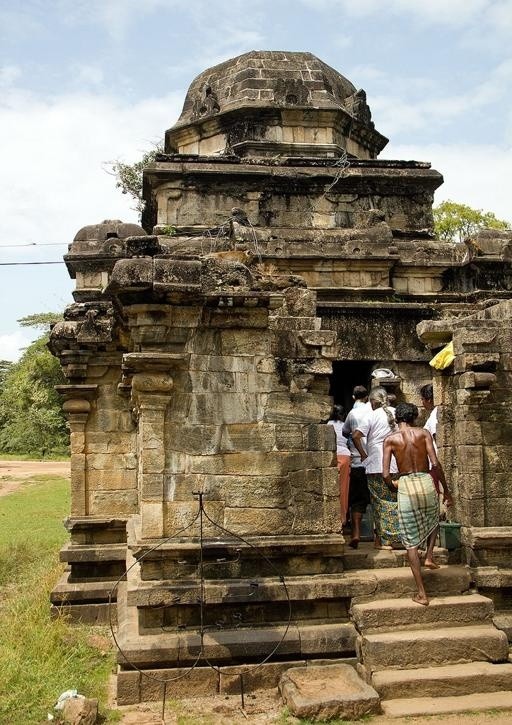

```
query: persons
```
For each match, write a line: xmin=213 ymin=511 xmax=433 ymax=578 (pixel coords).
xmin=326 ymin=383 xmax=453 ymax=605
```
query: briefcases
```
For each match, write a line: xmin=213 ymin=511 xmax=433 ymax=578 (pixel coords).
xmin=438 ymin=520 xmax=461 ymax=548
